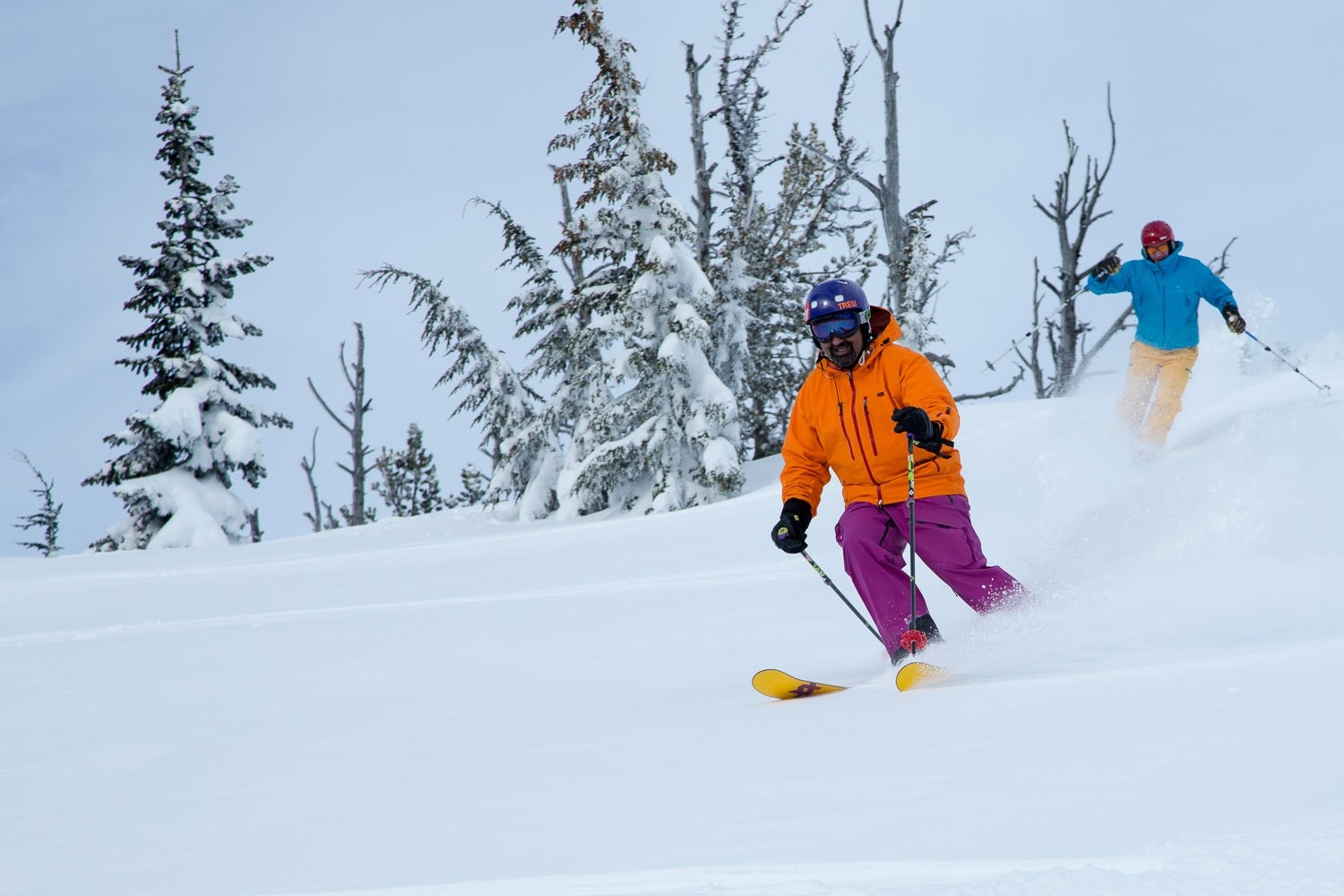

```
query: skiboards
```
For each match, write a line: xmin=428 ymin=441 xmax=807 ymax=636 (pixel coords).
xmin=749 ymin=661 xmax=933 ymax=701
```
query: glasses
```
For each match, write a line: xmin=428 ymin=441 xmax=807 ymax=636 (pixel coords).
xmin=809 ymin=311 xmax=859 ymax=343
xmin=1146 ymin=244 xmax=1168 ymax=254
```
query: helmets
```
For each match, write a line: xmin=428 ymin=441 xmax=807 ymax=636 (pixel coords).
xmin=1141 ymin=221 xmax=1175 ymax=252
xmin=805 ymin=279 xmax=872 ymax=353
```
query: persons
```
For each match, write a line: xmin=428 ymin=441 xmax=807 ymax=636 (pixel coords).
xmin=1086 ymin=220 xmax=1245 ymax=446
xmin=771 ymin=279 xmax=1025 ymax=668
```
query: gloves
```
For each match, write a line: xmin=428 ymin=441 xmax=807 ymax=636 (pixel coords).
xmin=1222 ymin=304 xmax=1245 ymax=335
xmin=1092 ymin=256 xmax=1120 ymax=282
xmin=771 ymin=497 xmax=811 ymax=553
xmin=891 ymin=407 xmax=943 ymax=451
